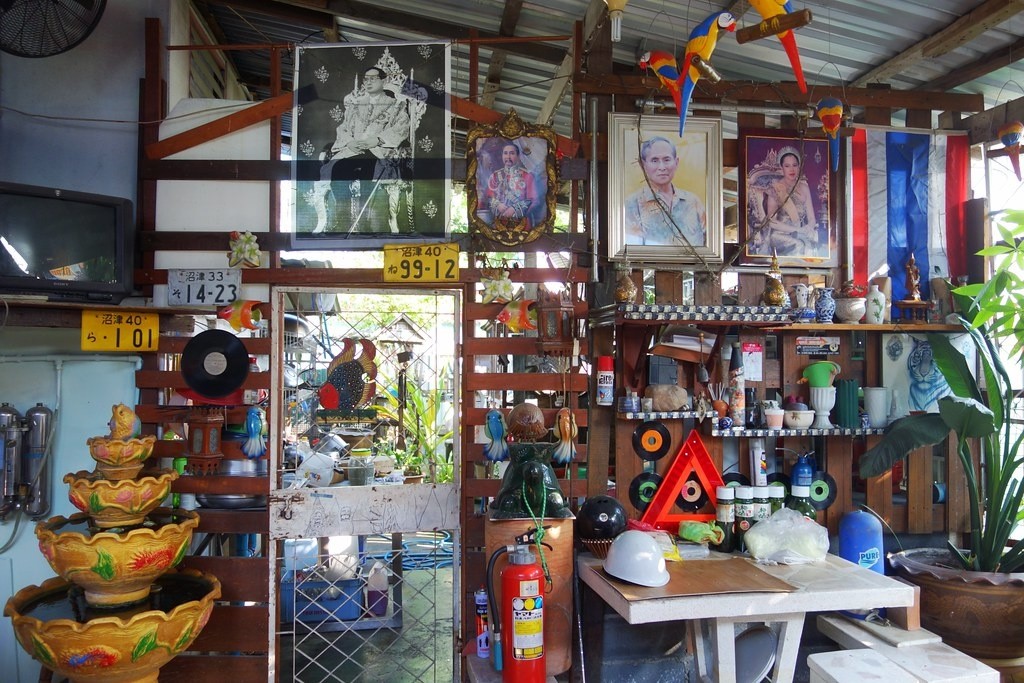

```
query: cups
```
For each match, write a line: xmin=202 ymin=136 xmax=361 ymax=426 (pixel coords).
xmin=641 ymin=398 xmax=652 ymax=413
xmin=765 ymin=409 xmax=784 ymax=430
xmin=927 ymin=299 xmax=941 ymax=323
xmin=863 ymin=387 xmax=888 ymax=428
xmin=835 ymin=378 xmax=858 ymax=429
xmin=810 ymin=386 xmax=835 ymax=429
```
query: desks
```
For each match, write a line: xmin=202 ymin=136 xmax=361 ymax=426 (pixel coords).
xmin=576 ymin=544 xmax=915 ymax=683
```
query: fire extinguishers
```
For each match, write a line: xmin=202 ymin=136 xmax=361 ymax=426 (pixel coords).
xmin=486 ymin=524 xmax=553 ymax=683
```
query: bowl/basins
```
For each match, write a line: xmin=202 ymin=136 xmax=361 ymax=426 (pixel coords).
xmin=784 ymin=410 xmax=815 ymax=429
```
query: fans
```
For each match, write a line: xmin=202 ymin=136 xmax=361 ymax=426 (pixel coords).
xmin=0 ymin=0 xmax=106 ymax=58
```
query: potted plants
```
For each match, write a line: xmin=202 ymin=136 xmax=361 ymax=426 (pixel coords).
xmin=832 ymin=280 xmax=867 ymax=325
xmin=859 ymin=208 xmax=1024 ymax=660
xmin=385 ymin=444 xmax=425 ymax=484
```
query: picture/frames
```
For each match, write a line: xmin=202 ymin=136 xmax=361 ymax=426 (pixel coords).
xmin=287 ymin=39 xmax=452 ymax=246
xmin=735 ymin=126 xmax=840 ymax=269
xmin=606 ymin=109 xmax=723 ymax=263
xmin=467 ymin=105 xmax=558 ymax=244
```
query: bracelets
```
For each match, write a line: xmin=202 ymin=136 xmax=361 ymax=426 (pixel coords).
xmin=377 ymin=137 xmax=385 ymax=144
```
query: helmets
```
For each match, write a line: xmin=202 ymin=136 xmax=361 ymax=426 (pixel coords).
xmin=602 ymin=529 xmax=671 ymax=588
xmin=575 ymin=494 xmax=626 ymax=540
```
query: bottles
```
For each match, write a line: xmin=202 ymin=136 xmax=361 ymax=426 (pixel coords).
xmin=348 ymin=448 xmax=375 ymax=487
xmin=763 ymin=272 xmax=785 ymax=305
xmin=597 ymin=355 xmax=614 ymax=405
xmin=475 ymin=584 xmax=489 ymax=658
xmin=368 ymin=561 xmax=387 ymax=615
xmin=865 ymin=284 xmax=886 ymax=323
xmin=789 ymin=283 xmax=816 ymax=323
xmin=728 ymin=342 xmax=746 ymax=431
xmin=715 ymin=484 xmax=817 ymax=552
xmin=815 ymin=287 xmax=836 ymax=323
xmin=243 ymin=358 xmax=259 ymax=404
xmin=745 ymin=387 xmax=760 ymax=428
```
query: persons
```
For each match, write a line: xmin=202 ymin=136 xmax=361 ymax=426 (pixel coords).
xmin=485 ymin=143 xmax=541 ymax=233
xmin=327 ymin=66 xmax=411 ymax=236
xmin=767 ymin=147 xmax=819 ymax=257
xmin=904 ymin=258 xmax=920 ymax=295
xmin=625 ymin=137 xmax=707 ymax=245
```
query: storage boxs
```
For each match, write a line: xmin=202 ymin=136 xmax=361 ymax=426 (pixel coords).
xmin=277 ymin=565 xmax=363 ymax=622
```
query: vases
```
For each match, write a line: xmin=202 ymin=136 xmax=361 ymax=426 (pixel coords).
xmin=834 ymin=377 xmax=860 ymax=429
xmin=865 ymin=284 xmax=886 ymax=324
xmin=808 ymin=385 xmax=837 ymax=430
xmin=814 ymin=287 xmax=836 ymax=324
